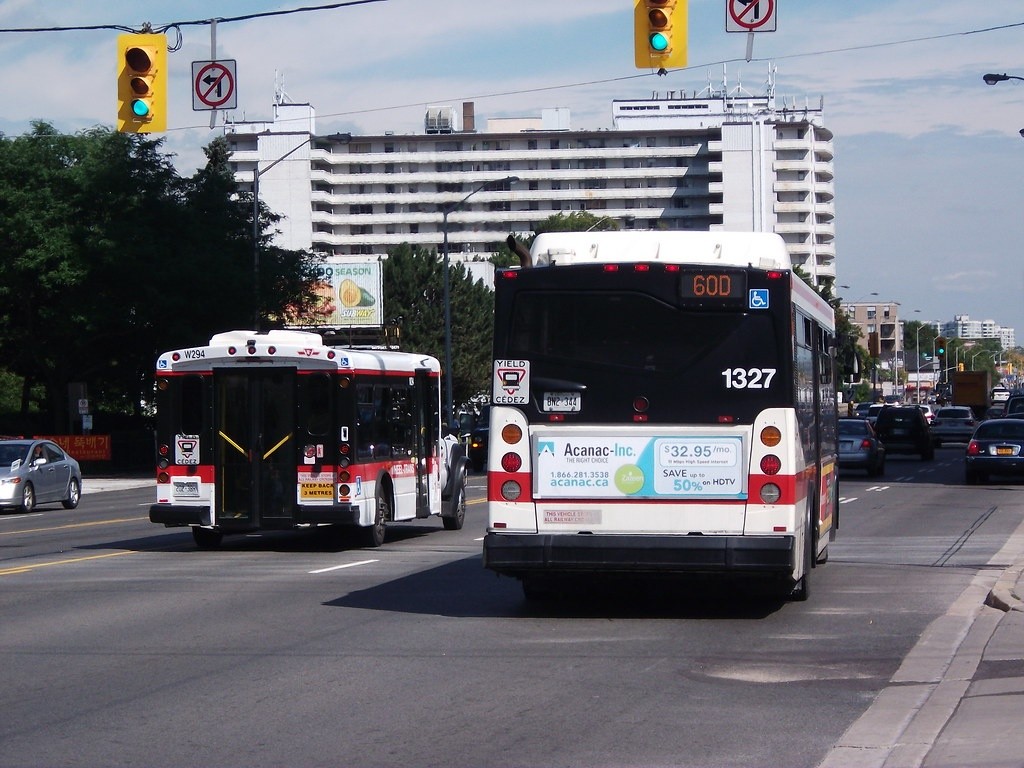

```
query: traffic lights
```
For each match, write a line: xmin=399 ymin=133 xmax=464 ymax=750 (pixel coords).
xmin=934 ymin=337 xmax=946 ymax=361
xmin=115 ymin=32 xmax=168 ymax=135
xmin=633 ymin=0 xmax=689 ymax=68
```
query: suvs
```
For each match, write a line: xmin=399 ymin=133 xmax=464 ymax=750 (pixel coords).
xmin=874 ymin=404 xmax=942 ymax=460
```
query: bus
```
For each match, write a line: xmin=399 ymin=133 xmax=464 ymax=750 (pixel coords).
xmin=145 ymin=325 xmax=470 ymax=549
xmin=482 ymin=228 xmax=862 ymax=600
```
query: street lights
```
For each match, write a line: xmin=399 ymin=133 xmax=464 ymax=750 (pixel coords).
xmin=964 ymin=342 xmax=983 ymax=365
xmin=955 ymin=341 xmax=975 ymax=371
xmin=933 ymin=327 xmax=956 ymax=390
xmin=253 ymin=131 xmax=354 ymax=330
xmin=442 ymin=174 xmax=522 ymax=432
xmin=971 ymin=349 xmax=991 ymax=370
xmin=872 ymin=300 xmax=900 ymax=404
xmin=916 ymin=319 xmax=943 ymax=403
xmin=894 ymin=309 xmax=923 ymax=395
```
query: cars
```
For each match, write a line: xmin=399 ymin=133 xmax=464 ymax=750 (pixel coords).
xmin=457 ymin=401 xmax=491 ymax=473
xmin=853 ymin=390 xmax=941 ymax=427
xmin=983 ymin=383 xmax=1024 ymax=418
xmin=964 ymin=418 xmax=1024 ymax=484
xmin=929 ymin=405 xmax=979 ymax=443
xmin=837 ymin=416 xmax=886 ymax=478
xmin=0 ymin=438 xmax=83 ymax=513
